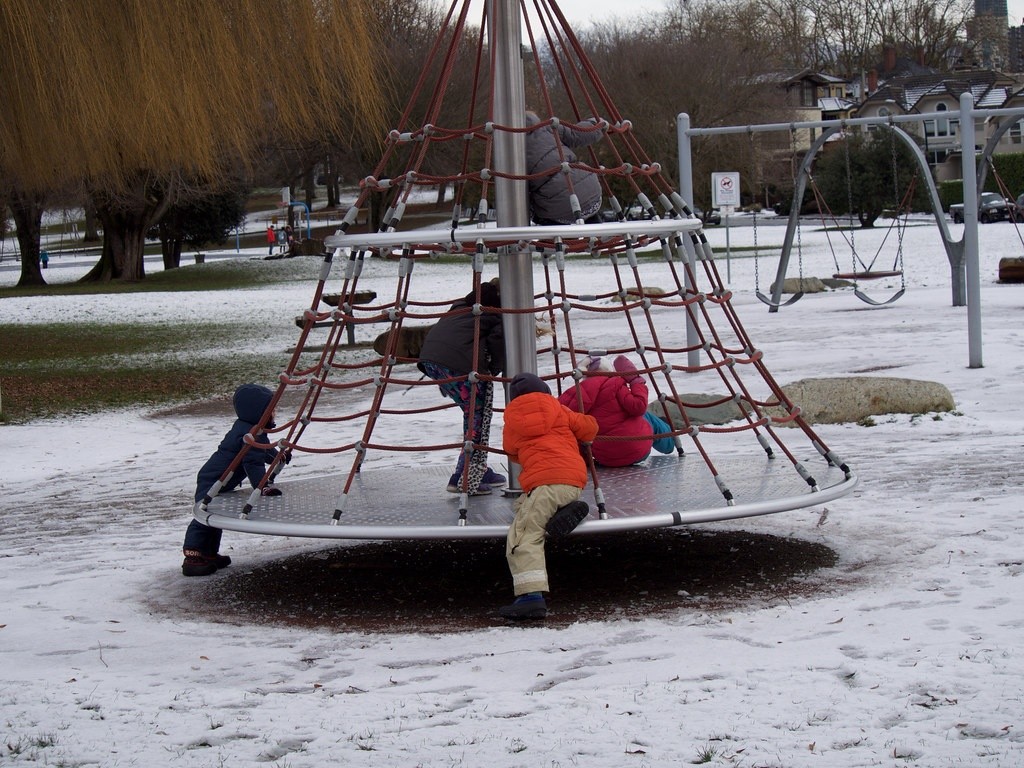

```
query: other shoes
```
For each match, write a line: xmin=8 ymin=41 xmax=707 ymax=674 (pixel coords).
xmin=182 ymin=553 xmax=231 ymax=576
xmin=585 ymin=211 xmax=603 ymax=224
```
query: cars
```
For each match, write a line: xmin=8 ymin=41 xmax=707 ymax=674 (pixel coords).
xmin=318 ymin=171 xmax=340 ymax=184
xmin=1013 ymin=193 xmax=1024 ymax=223
xmin=668 ymin=204 xmax=721 ymax=225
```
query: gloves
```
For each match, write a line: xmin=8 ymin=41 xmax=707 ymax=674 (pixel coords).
xmin=261 ymin=486 xmax=282 ymax=496
xmin=278 ymin=450 xmax=292 ymax=465
xmin=613 ymin=355 xmax=646 ymax=387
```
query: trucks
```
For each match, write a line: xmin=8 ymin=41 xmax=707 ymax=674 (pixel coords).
xmin=602 ymin=206 xmax=651 ymax=221
xmin=948 ymin=192 xmax=1015 ymax=224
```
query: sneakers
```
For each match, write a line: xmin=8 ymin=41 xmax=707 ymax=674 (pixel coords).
xmin=446 ymin=474 xmax=492 ymax=495
xmin=481 ymin=467 xmax=507 ymax=487
xmin=545 ymin=500 xmax=589 ymax=543
xmin=499 ymin=595 xmax=547 ymax=621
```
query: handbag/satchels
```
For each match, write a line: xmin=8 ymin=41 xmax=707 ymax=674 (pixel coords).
xmin=289 ymin=236 xmax=293 ymax=241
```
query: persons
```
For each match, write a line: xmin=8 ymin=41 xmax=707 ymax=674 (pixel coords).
xmin=278 ymin=227 xmax=287 ymax=254
xmin=182 ymin=383 xmax=291 ymax=578
xmin=497 ymin=374 xmax=598 ymax=621
xmin=38 ymin=248 xmax=49 ymax=269
xmin=268 ymin=226 xmax=275 ymax=255
xmin=525 ymin=111 xmax=609 ymax=225
xmin=554 ymin=353 xmax=676 ymax=468
xmin=286 ymin=225 xmax=294 ymax=250
xmin=416 ymin=276 xmax=551 ymax=497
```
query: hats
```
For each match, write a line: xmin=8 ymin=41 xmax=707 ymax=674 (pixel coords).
xmin=577 ymin=355 xmax=613 ymax=372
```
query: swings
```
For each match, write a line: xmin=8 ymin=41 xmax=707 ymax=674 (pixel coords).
xmin=990 ymin=159 xmax=1024 ymax=246
xmin=749 ymin=133 xmax=804 ymax=307
xmin=276 ymin=206 xmax=287 ymax=247
xmin=805 ymin=166 xmax=919 ymax=280
xmin=845 ymin=123 xmax=906 ymax=306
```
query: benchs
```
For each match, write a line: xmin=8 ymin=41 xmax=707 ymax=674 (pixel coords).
xmin=304 ymin=211 xmax=339 ymax=222
